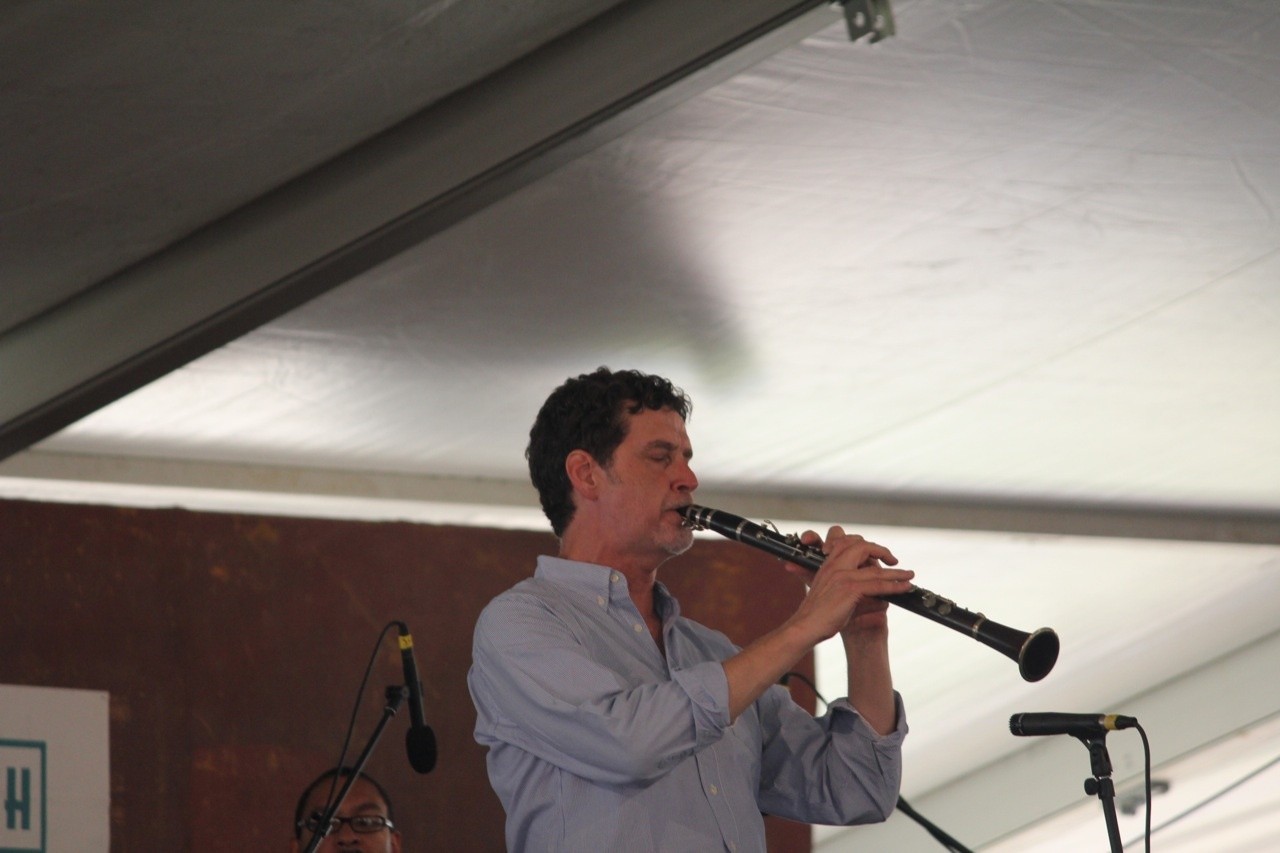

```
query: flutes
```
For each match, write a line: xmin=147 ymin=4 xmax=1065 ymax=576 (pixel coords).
xmin=677 ymin=504 xmax=1062 ymax=684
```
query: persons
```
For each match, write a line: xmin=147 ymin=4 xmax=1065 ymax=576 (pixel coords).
xmin=467 ymin=367 xmax=915 ymax=853
xmin=287 ymin=764 xmax=400 ymax=853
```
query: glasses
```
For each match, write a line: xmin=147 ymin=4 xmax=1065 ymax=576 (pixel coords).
xmin=296 ymin=811 xmax=395 ymax=835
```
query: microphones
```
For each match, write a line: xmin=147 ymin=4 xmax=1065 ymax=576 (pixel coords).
xmin=398 ymin=622 xmax=438 ymax=775
xmin=1009 ymin=712 xmax=1137 ymax=736
